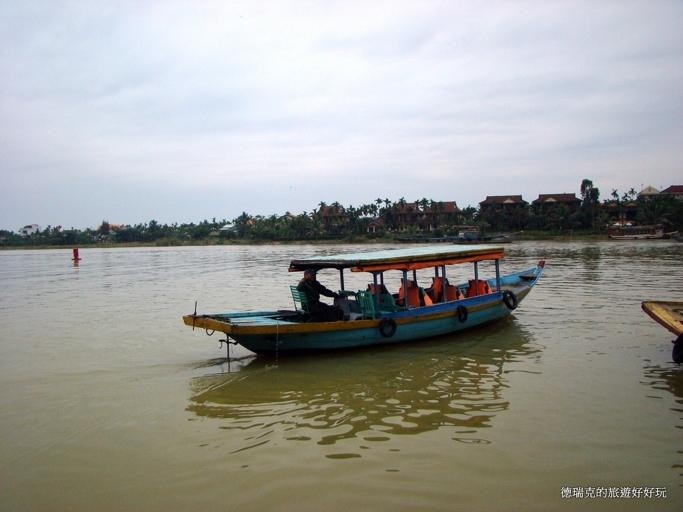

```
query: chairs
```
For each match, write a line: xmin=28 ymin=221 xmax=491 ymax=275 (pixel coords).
xmin=358 ymin=277 xmax=492 ymax=320
xmin=290 ymin=286 xmax=312 ymax=316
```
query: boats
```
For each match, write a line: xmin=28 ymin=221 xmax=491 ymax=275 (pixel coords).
xmin=639 ymin=300 xmax=682 ymax=364
xmin=451 ymin=230 xmax=522 ymax=244
xmin=609 ymin=227 xmax=678 ymax=240
xmin=183 ymin=244 xmax=548 ymax=354
xmin=182 ymin=316 xmax=532 ymax=428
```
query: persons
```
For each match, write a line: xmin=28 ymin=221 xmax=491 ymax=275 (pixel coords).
xmin=298 ymin=269 xmax=339 ymax=322
